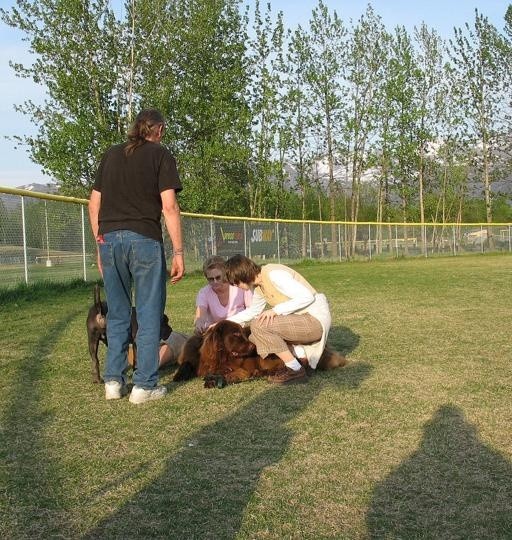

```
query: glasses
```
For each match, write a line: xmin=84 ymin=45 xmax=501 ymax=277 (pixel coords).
xmin=206 ymin=275 xmax=221 ymax=282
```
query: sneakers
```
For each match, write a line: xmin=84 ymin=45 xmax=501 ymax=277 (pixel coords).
xmin=128 ymin=385 xmax=167 ymax=404
xmin=105 ymin=381 xmax=122 ymax=400
xmin=268 ymin=365 xmax=306 ymax=382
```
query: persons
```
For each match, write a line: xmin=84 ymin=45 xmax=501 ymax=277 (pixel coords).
xmin=159 ymin=255 xmax=253 ymax=367
xmin=88 ymin=109 xmax=185 ymax=404
xmin=225 ymin=255 xmax=331 ymax=384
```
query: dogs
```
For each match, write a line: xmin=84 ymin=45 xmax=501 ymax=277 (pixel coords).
xmin=86 ymin=283 xmax=172 ymax=385
xmin=197 ymin=319 xmax=350 ymax=389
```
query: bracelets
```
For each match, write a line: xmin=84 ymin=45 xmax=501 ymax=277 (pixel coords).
xmin=173 ymin=247 xmax=183 ymax=255
